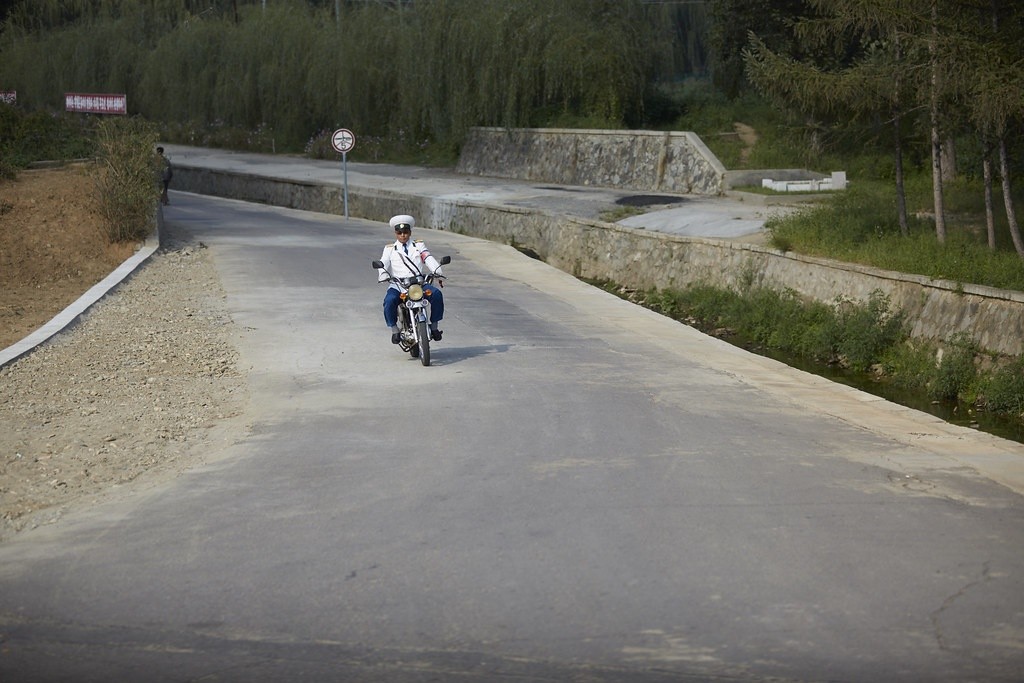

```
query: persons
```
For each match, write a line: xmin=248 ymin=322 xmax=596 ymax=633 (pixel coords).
xmin=155 ymin=147 xmax=172 ymax=205
xmin=378 ymin=215 xmax=443 ymax=345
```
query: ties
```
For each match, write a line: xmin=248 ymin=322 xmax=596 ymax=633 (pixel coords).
xmin=402 ymin=243 xmax=409 ymax=255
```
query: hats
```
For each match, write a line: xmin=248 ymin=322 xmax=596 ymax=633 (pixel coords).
xmin=389 ymin=215 xmax=415 ymax=231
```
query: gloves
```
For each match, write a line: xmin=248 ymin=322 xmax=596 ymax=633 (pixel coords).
xmin=434 ymin=271 xmax=443 ymax=279
xmin=378 ymin=273 xmax=390 ymax=284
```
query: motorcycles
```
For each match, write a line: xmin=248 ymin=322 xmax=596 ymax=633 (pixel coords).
xmin=371 ymin=256 xmax=452 ymax=366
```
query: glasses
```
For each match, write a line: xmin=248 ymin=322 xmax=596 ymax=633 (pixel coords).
xmin=394 ymin=230 xmax=410 ymax=235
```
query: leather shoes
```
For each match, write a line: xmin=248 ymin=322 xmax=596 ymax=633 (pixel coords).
xmin=432 ymin=329 xmax=443 ymax=341
xmin=392 ymin=332 xmax=400 ymax=344
